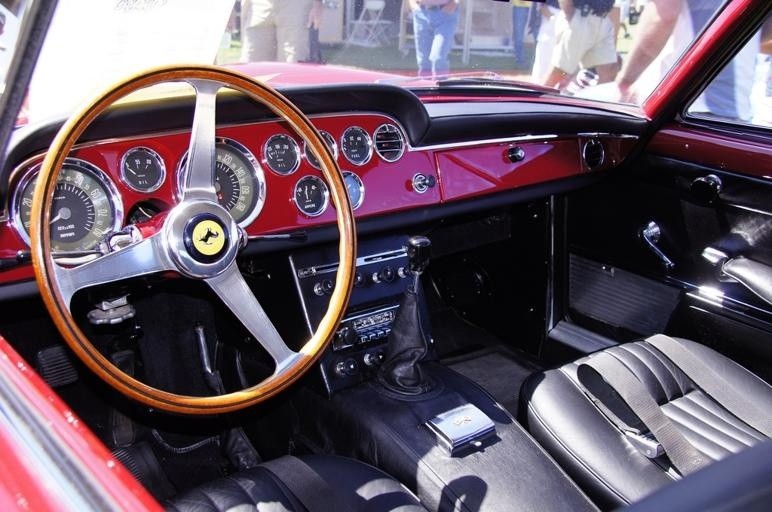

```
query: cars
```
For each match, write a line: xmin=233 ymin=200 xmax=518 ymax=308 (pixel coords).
xmin=0 ymin=4 xmax=32 ymax=130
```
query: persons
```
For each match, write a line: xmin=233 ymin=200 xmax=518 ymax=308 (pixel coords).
xmin=410 ymin=0 xmax=460 ymax=79
xmin=510 ymin=0 xmax=772 ymax=127
xmin=240 ymin=0 xmax=324 ymax=63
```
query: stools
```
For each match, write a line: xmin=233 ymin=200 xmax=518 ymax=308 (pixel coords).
xmin=165 ymin=453 xmax=433 ymax=512
xmin=516 ymin=330 xmax=771 ymax=510
